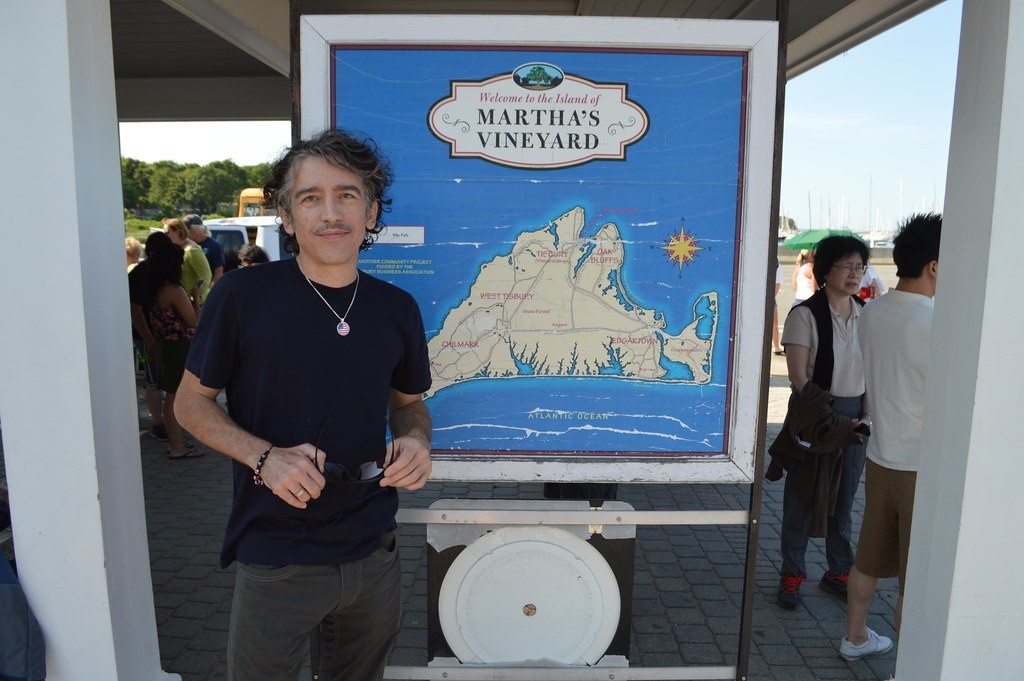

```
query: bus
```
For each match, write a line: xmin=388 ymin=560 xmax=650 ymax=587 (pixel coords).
xmin=238 ymin=188 xmax=279 ymax=217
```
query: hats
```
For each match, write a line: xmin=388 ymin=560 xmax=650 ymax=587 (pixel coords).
xmin=183 ymin=214 xmax=203 ymax=230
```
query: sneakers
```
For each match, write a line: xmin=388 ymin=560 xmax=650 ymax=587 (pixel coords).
xmin=819 ymin=570 xmax=849 ymax=603
xmin=150 ymin=423 xmax=169 ymax=441
xmin=840 ymin=626 xmax=893 ymax=662
xmin=776 ymin=576 xmax=806 ymax=610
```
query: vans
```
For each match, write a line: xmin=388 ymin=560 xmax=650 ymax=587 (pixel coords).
xmin=201 ymin=216 xmax=281 ymax=262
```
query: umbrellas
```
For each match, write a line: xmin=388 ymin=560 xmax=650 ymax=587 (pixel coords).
xmin=783 ymin=228 xmax=870 ymax=251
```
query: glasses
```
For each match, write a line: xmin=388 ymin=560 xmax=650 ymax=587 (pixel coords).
xmin=314 ymin=417 xmax=394 ymax=483
xmin=165 ymin=232 xmax=170 ymax=235
xmin=832 ymin=265 xmax=868 ymax=274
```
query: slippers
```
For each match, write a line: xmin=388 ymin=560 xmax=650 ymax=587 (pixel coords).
xmin=166 ymin=443 xmax=194 ymax=454
xmin=169 ymin=449 xmax=205 ymax=459
xmin=773 ymin=350 xmax=786 ymax=356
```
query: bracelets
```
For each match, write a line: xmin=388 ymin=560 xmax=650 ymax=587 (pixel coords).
xmin=255 ymin=444 xmax=274 ymax=486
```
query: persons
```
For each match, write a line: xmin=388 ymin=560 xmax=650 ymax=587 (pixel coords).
xmin=839 ymin=213 xmax=944 ymax=681
xmin=171 ymin=131 xmax=434 ymax=681
xmin=126 ymin=213 xmax=269 ymax=458
xmin=770 ymin=249 xmax=884 ymax=356
xmin=767 ymin=235 xmax=876 ymax=609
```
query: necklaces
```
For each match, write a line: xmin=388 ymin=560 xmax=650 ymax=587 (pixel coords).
xmin=296 ymin=257 xmax=360 ymax=336
